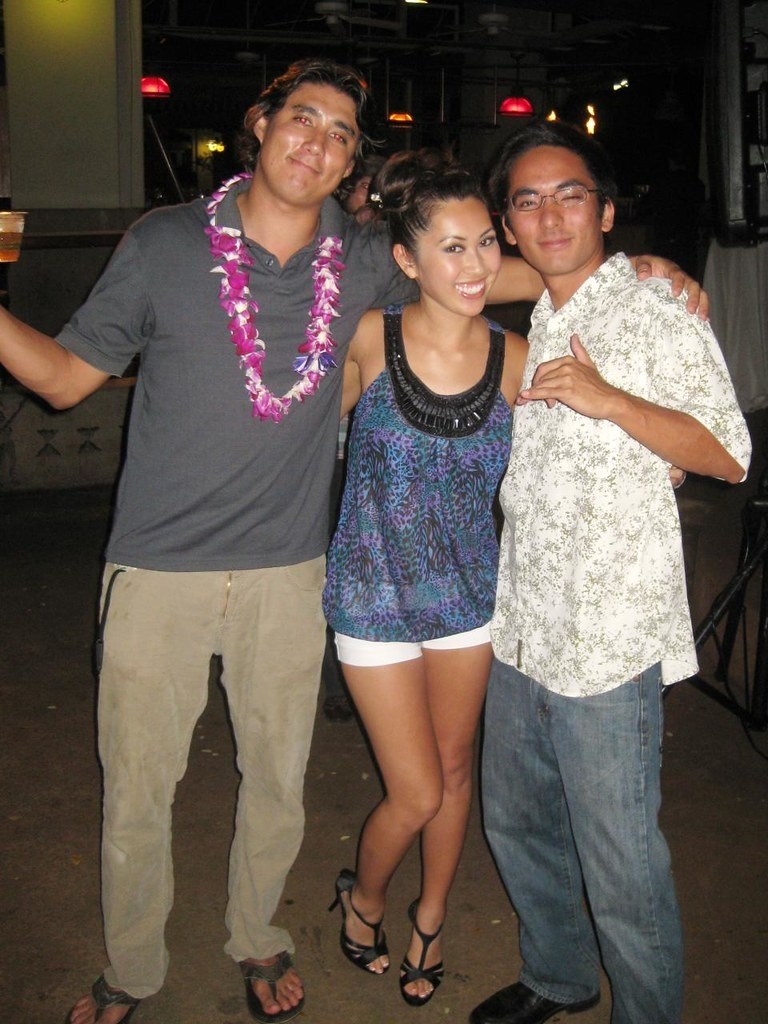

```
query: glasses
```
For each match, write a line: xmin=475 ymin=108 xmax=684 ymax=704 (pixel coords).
xmin=503 ymin=185 xmax=602 ymax=212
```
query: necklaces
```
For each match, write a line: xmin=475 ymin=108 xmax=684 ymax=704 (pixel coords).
xmin=205 ymin=174 xmax=347 ymax=422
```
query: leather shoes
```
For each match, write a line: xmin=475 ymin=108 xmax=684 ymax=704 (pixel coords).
xmin=469 ymin=978 xmax=601 ymax=1024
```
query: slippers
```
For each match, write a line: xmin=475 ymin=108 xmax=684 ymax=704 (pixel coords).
xmin=65 ymin=973 xmax=140 ymax=1024
xmin=236 ymin=950 xmax=305 ymax=1024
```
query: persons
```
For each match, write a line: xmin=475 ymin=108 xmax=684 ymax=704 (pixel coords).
xmin=322 ymin=173 xmax=696 ymax=1007
xmin=468 ymin=129 xmax=752 ymax=1024
xmin=0 ymin=58 xmax=712 ymax=1023
xmin=332 ymin=155 xmax=389 ymax=225
xmin=439 ymin=123 xmax=540 ymax=177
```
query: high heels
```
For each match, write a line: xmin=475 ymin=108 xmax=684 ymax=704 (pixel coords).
xmin=398 ymin=898 xmax=444 ymax=1007
xmin=328 ymin=868 xmax=391 ymax=975
